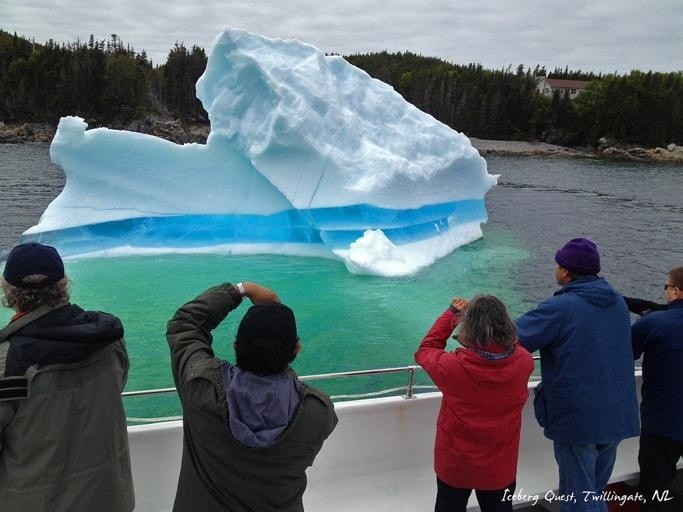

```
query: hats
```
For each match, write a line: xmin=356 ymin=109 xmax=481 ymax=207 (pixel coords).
xmin=1 ymin=241 xmax=66 ymax=292
xmin=553 ymin=236 xmax=601 ymax=276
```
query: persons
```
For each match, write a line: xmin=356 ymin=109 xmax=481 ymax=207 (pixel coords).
xmin=631 ymin=265 xmax=683 ymax=511
xmin=512 ymin=237 xmax=641 ymax=511
xmin=0 ymin=242 xmax=137 ymax=511
xmin=164 ymin=280 xmax=339 ymax=511
xmin=413 ymin=293 xmax=535 ymax=511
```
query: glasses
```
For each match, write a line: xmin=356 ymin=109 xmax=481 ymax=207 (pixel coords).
xmin=662 ymin=283 xmax=677 ymax=292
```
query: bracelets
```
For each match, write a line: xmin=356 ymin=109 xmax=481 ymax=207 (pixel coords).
xmin=236 ymin=282 xmax=246 ymax=297
